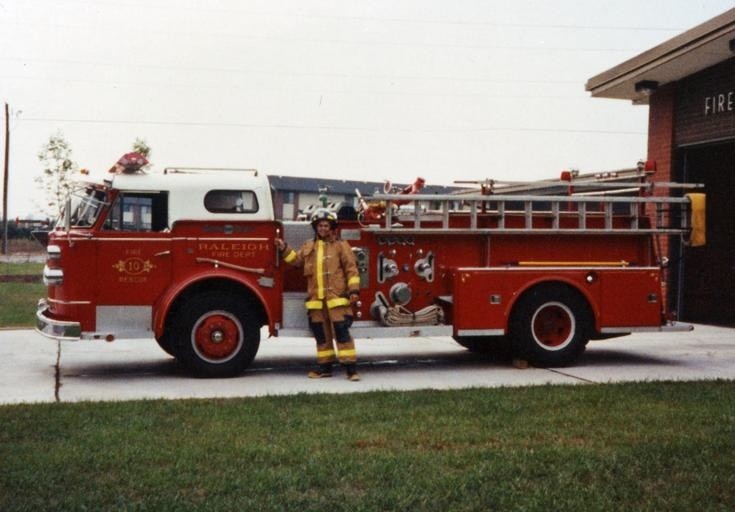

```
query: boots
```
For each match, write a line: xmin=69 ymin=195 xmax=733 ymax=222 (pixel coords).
xmin=344 ymin=361 xmax=360 ymax=382
xmin=308 ymin=362 xmax=334 ymax=378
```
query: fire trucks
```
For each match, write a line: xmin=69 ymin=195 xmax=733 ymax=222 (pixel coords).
xmin=35 ymin=153 xmax=709 ymax=379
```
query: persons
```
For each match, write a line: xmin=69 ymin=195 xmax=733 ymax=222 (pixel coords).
xmin=275 ymin=209 xmax=363 ymax=383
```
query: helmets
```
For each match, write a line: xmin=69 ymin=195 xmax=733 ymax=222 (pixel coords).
xmin=311 ymin=208 xmax=337 ymax=227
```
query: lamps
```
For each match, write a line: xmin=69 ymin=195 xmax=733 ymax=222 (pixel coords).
xmin=635 ymin=79 xmax=658 ymax=95
